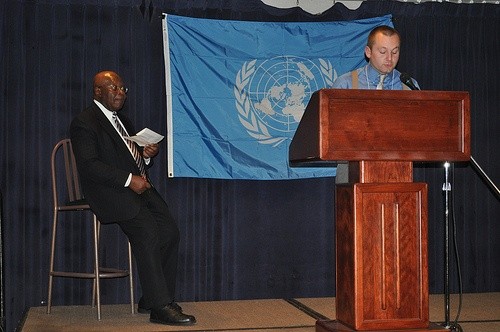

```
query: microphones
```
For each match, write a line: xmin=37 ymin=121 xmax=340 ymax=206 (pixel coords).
xmin=399 ymin=72 xmax=419 ymax=90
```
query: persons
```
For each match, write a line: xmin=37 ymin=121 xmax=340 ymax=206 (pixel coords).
xmin=70 ymin=71 xmax=197 ymax=326
xmin=331 ymin=25 xmax=421 ymax=90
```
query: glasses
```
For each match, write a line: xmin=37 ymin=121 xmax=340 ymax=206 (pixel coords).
xmin=98 ymin=83 xmax=129 ymax=94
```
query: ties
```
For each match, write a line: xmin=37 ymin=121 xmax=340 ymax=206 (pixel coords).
xmin=112 ymin=111 xmax=146 ymax=176
xmin=375 ymin=74 xmax=388 ymax=90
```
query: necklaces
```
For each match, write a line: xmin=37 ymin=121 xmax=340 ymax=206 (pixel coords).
xmin=365 ymin=65 xmax=394 ymax=90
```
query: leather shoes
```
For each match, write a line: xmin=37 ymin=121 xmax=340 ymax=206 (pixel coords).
xmin=150 ymin=302 xmax=197 ymax=326
xmin=137 ymin=297 xmax=182 ymax=314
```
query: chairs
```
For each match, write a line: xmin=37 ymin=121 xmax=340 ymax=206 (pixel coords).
xmin=46 ymin=139 xmax=135 ymax=322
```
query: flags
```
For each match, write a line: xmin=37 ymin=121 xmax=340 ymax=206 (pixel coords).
xmin=161 ymin=14 xmax=398 ymax=180
